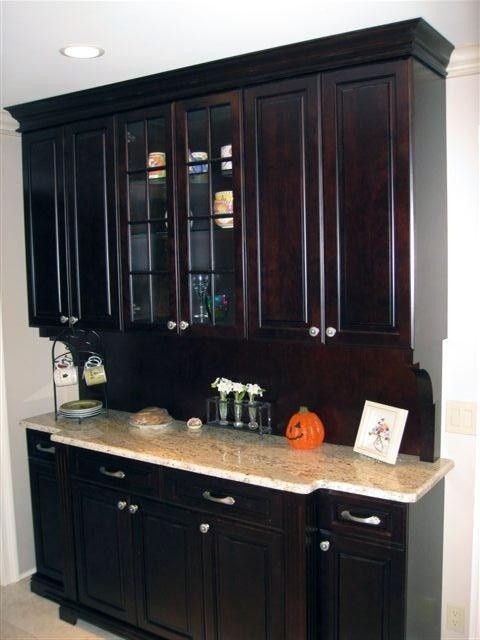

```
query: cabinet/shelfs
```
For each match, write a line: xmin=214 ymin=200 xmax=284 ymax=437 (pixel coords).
xmin=26 ymin=428 xmax=75 ymax=610
xmin=119 ymin=92 xmax=246 ymax=338
xmin=24 ymin=117 xmax=120 ymax=330
xmin=76 ymin=447 xmax=205 ymax=640
xmin=246 ymin=59 xmax=453 ymax=344
xmin=316 ymin=466 xmax=447 ymax=640
xmin=206 ymin=474 xmax=323 ymax=640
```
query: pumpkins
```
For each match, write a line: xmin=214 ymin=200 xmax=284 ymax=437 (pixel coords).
xmin=286 ymin=407 xmax=325 ymax=451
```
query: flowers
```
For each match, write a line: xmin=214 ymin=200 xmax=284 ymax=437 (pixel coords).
xmin=230 ymin=381 xmax=247 ymax=404
xmin=212 ymin=375 xmax=232 ymax=400
xmin=244 ymin=383 xmax=265 ymax=403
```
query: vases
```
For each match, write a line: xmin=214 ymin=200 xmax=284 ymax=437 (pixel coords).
xmin=247 ymin=402 xmax=258 ymax=430
xmin=218 ymin=400 xmax=228 ymax=426
xmin=234 ymin=401 xmax=244 ymax=428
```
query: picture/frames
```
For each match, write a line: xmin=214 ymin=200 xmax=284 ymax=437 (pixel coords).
xmin=353 ymin=400 xmax=410 ymax=465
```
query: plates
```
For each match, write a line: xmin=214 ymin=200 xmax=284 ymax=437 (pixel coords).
xmin=164 ymin=209 xmax=194 ymax=231
xmin=58 ymin=398 xmax=102 ymax=420
xmin=128 ymin=420 xmax=175 ymax=432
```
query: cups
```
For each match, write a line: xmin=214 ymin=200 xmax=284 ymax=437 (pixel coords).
xmin=52 ymin=357 xmax=79 ymax=387
xmin=191 ymin=274 xmax=211 ymax=318
xmin=187 ymin=151 xmax=211 ymax=184
xmin=82 ymin=356 xmax=108 ymax=387
xmin=148 ymin=151 xmax=168 ymax=186
xmin=220 ymin=144 xmax=233 ymax=177
